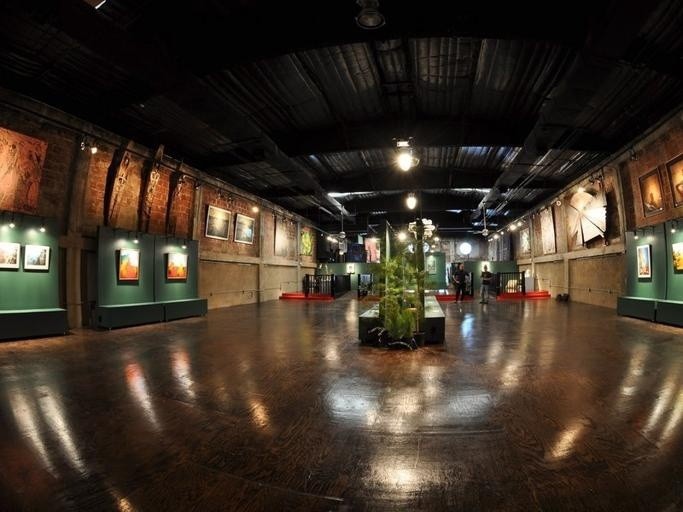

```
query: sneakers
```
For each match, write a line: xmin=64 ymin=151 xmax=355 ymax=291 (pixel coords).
xmin=479 ymin=300 xmax=488 ymax=304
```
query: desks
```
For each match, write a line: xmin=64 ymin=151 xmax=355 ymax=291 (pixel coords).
xmin=520 ymin=277 xmax=534 ymax=292
xmin=617 ymin=296 xmax=683 ymax=328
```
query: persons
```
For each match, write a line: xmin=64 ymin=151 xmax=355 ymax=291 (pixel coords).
xmin=452 ymin=263 xmax=470 ymax=302
xmin=478 ymin=265 xmax=494 ymax=305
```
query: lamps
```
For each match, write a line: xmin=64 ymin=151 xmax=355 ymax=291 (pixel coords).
xmin=634 ymin=219 xmax=678 ymax=240
xmin=339 ymin=206 xmax=346 ymax=238
xmin=80 ymin=142 xmax=98 ymax=154
xmin=482 ymin=203 xmax=488 ymax=236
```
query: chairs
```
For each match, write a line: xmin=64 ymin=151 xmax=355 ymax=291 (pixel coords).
xmin=505 ymin=280 xmax=517 ymax=293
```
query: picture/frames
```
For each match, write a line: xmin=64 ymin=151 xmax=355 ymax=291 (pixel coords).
xmin=664 ymin=152 xmax=683 ymax=208
xmin=0 ymin=242 xmax=21 ymax=271
xmin=205 ymin=205 xmax=231 ymax=241
xmin=637 ymin=167 xmax=666 ymax=218
xmin=23 ymin=245 xmax=50 ymax=271
xmin=166 ymin=253 xmax=189 ymax=280
xmin=671 ymin=242 xmax=683 ymax=274
xmin=119 ymin=249 xmax=141 ymax=281
xmin=233 ymin=213 xmax=255 ymax=244
xmin=637 ymin=244 xmax=652 ymax=278
